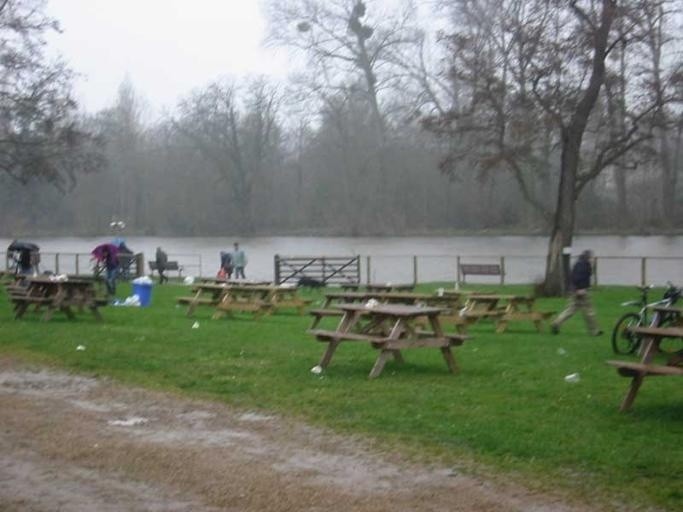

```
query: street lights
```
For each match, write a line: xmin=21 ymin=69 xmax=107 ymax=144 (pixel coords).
xmin=111 ymin=219 xmax=126 ymax=277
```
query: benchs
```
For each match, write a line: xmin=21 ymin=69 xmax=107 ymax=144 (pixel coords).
xmin=176 ymin=293 xmax=309 ymax=324
xmin=604 ymin=358 xmax=682 ymax=383
xmin=9 ymin=287 xmax=109 ymax=321
xmin=313 ymin=309 xmax=345 ymax=317
xmin=452 ymin=309 xmax=557 ymax=334
xmin=305 ymin=328 xmax=466 ymax=353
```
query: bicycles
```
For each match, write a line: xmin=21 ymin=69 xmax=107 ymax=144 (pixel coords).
xmin=610 ymin=279 xmax=681 ymax=358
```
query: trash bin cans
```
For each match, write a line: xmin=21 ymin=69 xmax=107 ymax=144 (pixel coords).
xmin=131 ymin=283 xmax=153 ymax=307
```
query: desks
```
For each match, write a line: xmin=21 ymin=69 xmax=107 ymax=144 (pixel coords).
xmin=9 ymin=275 xmax=110 ymax=322
xmin=617 ymin=302 xmax=682 ymax=414
xmin=179 ymin=279 xmax=310 ymax=324
xmin=314 ymin=303 xmax=466 ymax=380
xmin=312 ymin=285 xmax=452 ymax=325
xmin=452 ymin=292 xmax=544 ymax=332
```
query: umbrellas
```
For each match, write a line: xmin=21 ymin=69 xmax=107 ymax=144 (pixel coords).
xmin=112 ymin=238 xmax=127 ymax=247
xmin=8 ymin=240 xmax=39 ymax=251
xmin=91 ymin=242 xmax=118 ymax=257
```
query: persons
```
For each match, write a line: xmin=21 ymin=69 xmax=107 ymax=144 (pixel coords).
xmin=118 ymin=242 xmax=134 ymax=281
xmin=550 ymin=249 xmax=604 ymax=337
xmin=102 ymin=248 xmax=120 ymax=295
xmin=32 ymin=249 xmax=41 ymax=275
xmin=231 ymin=242 xmax=248 ymax=279
xmin=219 ymin=251 xmax=234 ymax=279
xmin=15 ymin=249 xmax=34 ymax=275
xmin=155 ymin=246 xmax=169 ymax=284
